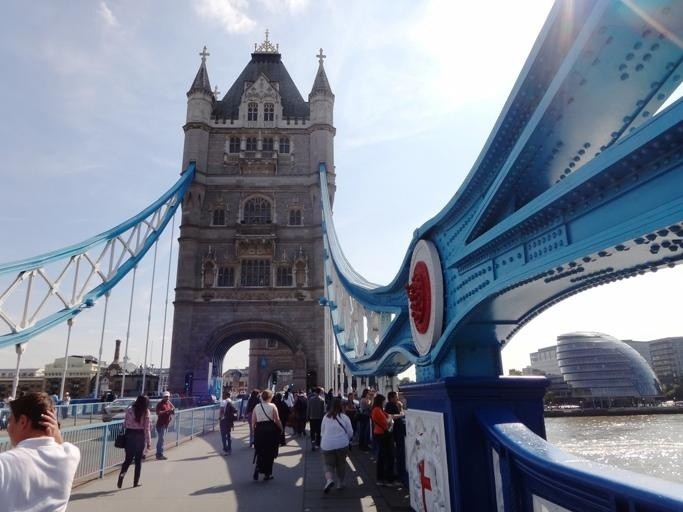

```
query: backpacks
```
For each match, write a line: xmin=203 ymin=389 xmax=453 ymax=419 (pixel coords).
xmin=222 ymin=399 xmax=237 ymax=426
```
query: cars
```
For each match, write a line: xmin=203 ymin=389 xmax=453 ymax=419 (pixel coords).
xmin=197 ymin=394 xmax=217 ymax=406
xmin=112 ymin=399 xmax=176 ymax=436
xmin=102 ymin=399 xmax=137 ymax=421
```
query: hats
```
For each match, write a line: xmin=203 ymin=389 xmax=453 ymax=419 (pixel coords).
xmin=162 ymin=390 xmax=171 ymax=397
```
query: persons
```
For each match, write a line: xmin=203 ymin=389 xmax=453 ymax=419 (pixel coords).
xmin=272 ymin=393 xmax=287 ymax=434
xmin=371 ymin=395 xmax=403 ymax=486
xmin=307 ymin=387 xmax=325 ymax=450
xmin=116 ymin=394 xmax=152 ymax=488
xmin=321 ymin=399 xmax=355 ymax=493
xmin=337 ymin=394 xmax=342 ymax=401
xmin=327 ymin=389 xmax=333 ymax=404
xmin=294 ymin=392 xmax=308 ymax=436
xmin=218 ymin=392 xmax=233 ymax=453
xmin=246 ymin=392 xmax=261 ymax=447
xmin=51 ymin=392 xmax=60 ymax=403
xmin=344 ymin=394 xmax=362 ymax=428
xmin=0 ymin=393 xmax=80 ymax=512
xmin=62 ymin=392 xmax=71 ymax=418
xmin=386 ymin=391 xmax=406 ymax=454
xmin=283 ymin=391 xmax=293 ymax=417
xmin=359 ymin=389 xmax=374 ymax=450
xmin=251 ymin=389 xmax=283 ymax=481
xmin=155 ymin=391 xmax=174 ymax=460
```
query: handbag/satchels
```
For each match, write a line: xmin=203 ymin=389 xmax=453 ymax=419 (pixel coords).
xmin=115 ymin=426 xmax=126 ymax=449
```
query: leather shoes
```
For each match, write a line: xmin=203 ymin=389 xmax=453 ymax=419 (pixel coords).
xmin=263 ymin=475 xmax=274 ymax=481
xmin=253 ymin=473 xmax=259 ymax=480
xmin=118 ymin=473 xmax=125 ymax=488
xmin=134 ymin=482 xmax=143 ymax=487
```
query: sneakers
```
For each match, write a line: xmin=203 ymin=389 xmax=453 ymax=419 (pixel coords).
xmin=311 ymin=439 xmax=316 ymax=444
xmin=375 ymin=479 xmax=385 ymax=486
xmin=158 ymin=456 xmax=168 ymax=460
xmin=228 ymin=449 xmax=234 ymax=456
xmin=322 ymin=478 xmax=336 ymax=492
xmin=221 ymin=450 xmax=228 ymax=456
xmin=336 ymin=481 xmax=345 ymax=489
xmin=386 ymin=481 xmax=403 ymax=487
xmin=315 ymin=445 xmax=319 ymax=449
